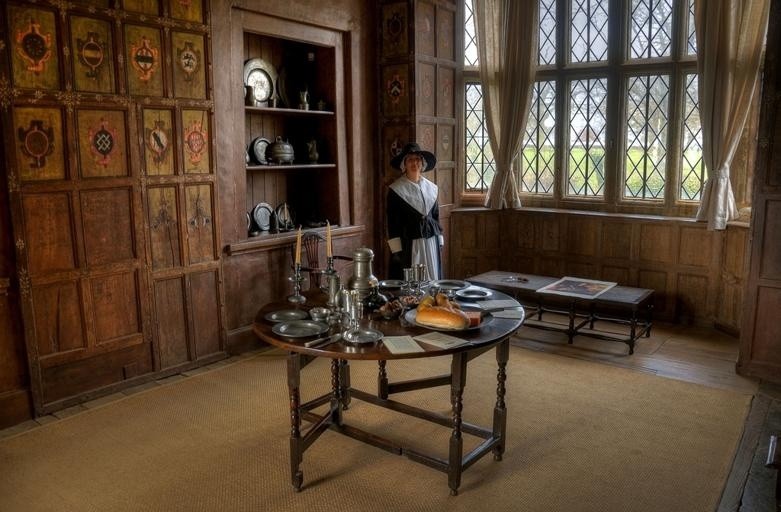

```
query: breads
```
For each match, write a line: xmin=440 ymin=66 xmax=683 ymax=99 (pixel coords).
xmin=417 ymin=296 xmax=435 ymax=311
xmin=416 ymin=306 xmax=471 ymax=330
xmin=436 ymin=293 xmax=452 ymax=309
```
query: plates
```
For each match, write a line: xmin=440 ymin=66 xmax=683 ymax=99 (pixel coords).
xmin=278 ymin=65 xmax=291 ymax=108
xmin=263 ymin=279 xmax=495 ymax=347
xmin=254 ymin=202 xmax=275 ymax=230
xmin=243 ymin=57 xmax=282 ymax=107
xmin=254 ymin=137 xmax=275 ymax=165
xmin=278 ymin=203 xmax=295 ymax=226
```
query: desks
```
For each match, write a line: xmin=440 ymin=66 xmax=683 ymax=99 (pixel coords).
xmin=465 ymin=269 xmax=656 ymax=356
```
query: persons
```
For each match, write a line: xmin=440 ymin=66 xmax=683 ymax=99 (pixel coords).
xmin=386 ymin=143 xmax=445 ymax=283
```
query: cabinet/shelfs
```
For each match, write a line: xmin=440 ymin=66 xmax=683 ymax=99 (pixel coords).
xmin=242 ymin=29 xmax=342 ymax=242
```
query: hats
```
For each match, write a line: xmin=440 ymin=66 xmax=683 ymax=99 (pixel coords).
xmin=390 ymin=142 xmax=436 ymax=172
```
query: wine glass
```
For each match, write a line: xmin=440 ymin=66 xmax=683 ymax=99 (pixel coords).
xmin=412 ymin=263 xmax=427 ymax=297
xmin=403 ymin=268 xmax=415 ymax=295
